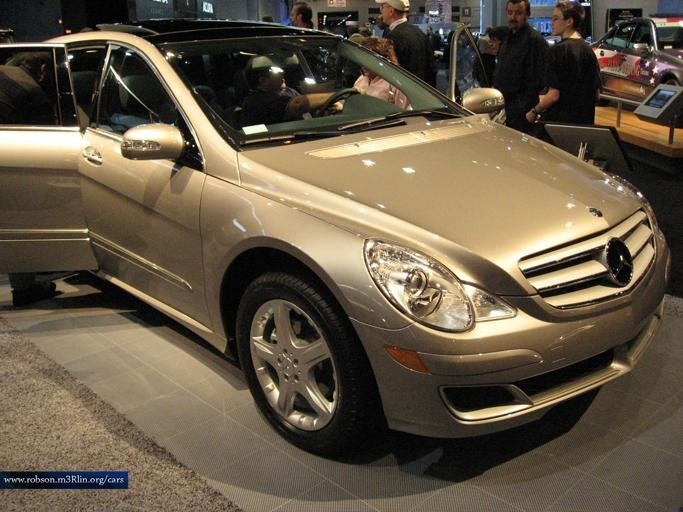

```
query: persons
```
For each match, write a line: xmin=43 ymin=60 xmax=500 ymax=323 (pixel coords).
xmin=241 ymin=55 xmax=342 ymax=125
xmin=425 ymin=24 xmax=509 ymax=100
xmin=0 ymin=50 xmax=56 ymax=307
xmin=349 ymin=34 xmax=412 ymax=111
xmin=492 ymin=0 xmax=548 ymax=140
xmin=525 ymin=0 xmax=602 ymax=145
xmin=287 ymin=2 xmax=312 ymax=30
xmin=374 ymin=0 xmax=435 ymax=90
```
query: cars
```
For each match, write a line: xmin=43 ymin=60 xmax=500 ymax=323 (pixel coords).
xmin=586 ymin=17 xmax=682 ymax=91
xmin=0 ymin=17 xmax=674 ymax=458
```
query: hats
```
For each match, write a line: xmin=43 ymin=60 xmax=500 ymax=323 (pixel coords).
xmin=374 ymin=1 xmax=411 ymax=12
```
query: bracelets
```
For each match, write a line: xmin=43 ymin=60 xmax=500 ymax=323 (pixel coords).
xmin=531 ymin=108 xmax=537 ymax=115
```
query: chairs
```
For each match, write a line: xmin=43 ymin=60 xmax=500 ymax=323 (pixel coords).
xmin=72 ymin=70 xmax=95 ymax=103
xmin=112 ymin=76 xmax=175 ymax=128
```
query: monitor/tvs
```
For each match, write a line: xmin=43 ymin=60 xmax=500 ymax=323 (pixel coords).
xmin=633 ymin=83 xmax=683 ymax=124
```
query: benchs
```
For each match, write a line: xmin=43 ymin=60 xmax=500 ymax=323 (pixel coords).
xmin=595 ymin=95 xmax=683 ymax=176
